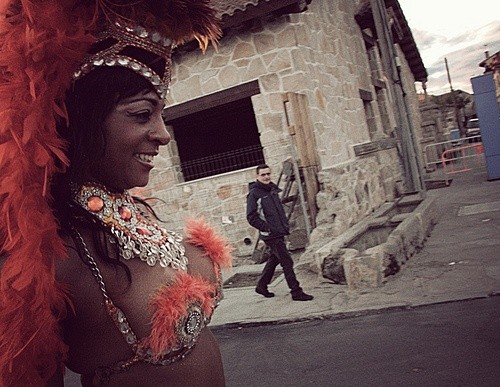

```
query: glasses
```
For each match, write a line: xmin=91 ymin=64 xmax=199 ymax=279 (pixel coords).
xmin=259 ymin=173 xmax=271 ymax=176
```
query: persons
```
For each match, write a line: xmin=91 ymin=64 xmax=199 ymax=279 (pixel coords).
xmin=246 ymin=165 xmax=315 ymax=301
xmin=0 ymin=0 xmax=229 ymax=387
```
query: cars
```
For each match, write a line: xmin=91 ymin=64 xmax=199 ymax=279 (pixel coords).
xmin=466 ymin=118 xmax=480 ymax=136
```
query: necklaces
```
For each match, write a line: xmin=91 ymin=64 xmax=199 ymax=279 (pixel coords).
xmin=64 ymin=180 xmax=190 ymax=274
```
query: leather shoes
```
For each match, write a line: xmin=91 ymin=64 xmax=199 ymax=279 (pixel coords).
xmin=293 ymin=292 xmax=313 ymax=300
xmin=255 ymin=288 xmax=274 ymax=297
